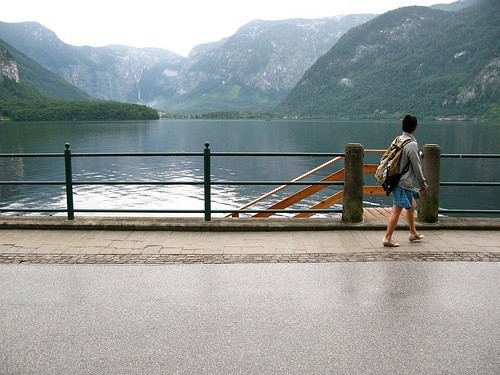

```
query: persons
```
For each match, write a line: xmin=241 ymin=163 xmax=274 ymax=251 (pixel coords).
xmin=374 ymin=114 xmax=430 ymax=247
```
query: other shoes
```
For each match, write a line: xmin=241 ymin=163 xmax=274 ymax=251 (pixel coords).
xmin=383 ymin=238 xmax=400 ymax=247
xmin=409 ymin=233 xmax=424 ymax=242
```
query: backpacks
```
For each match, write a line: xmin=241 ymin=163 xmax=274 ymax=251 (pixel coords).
xmin=375 ymin=142 xmax=404 ymax=196
